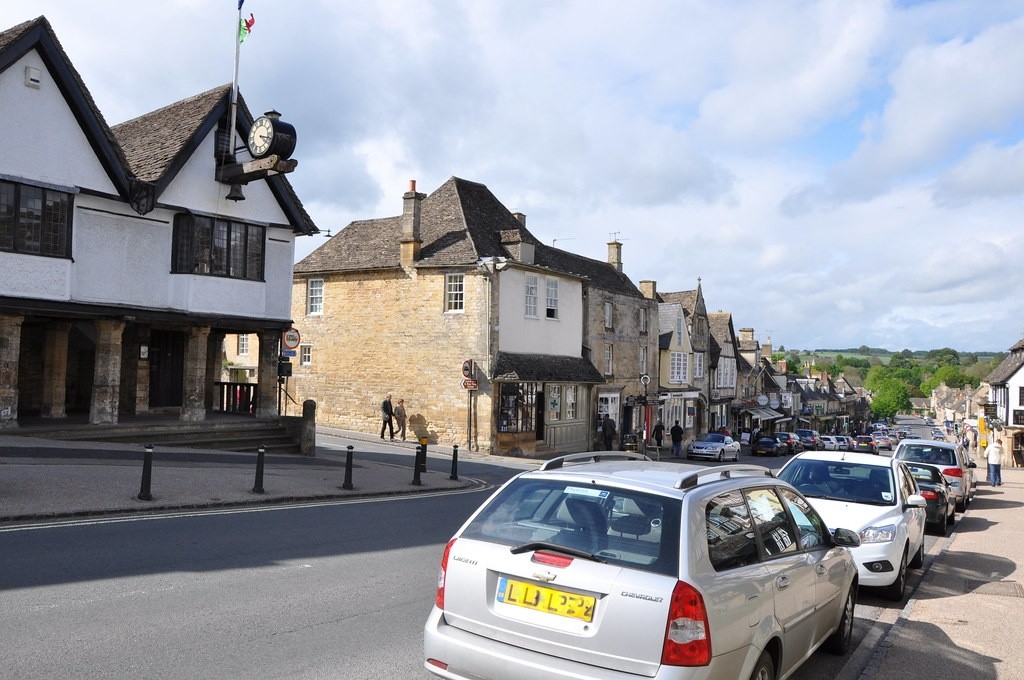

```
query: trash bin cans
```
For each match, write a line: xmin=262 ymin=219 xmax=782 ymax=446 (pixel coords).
xmin=623 ymin=433 xmax=639 ymax=460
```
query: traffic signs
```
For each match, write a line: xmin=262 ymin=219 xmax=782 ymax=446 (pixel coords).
xmin=461 ymin=380 xmax=478 ymax=389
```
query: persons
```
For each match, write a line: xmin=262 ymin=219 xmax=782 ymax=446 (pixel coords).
xmin=651 ymin=419 xmax=665 ymax=452
xmin=830 ymin=424 xmax=877 ymax=437
xmin=753 ymin=425 xmax=760 ymax=440
xmin=983 ymin=439 xmax=1004 ymax=487
xmin=963 ymin=436 xmax=970 ymax=452
xmin=602 ymin=414 xmax=616 ymax=451
xmin=946 ymin=421 xmax=959 ymax=435
xmin=671 ymin=420 xmax=684 ymax=456
xmin=393 ymin=398 xmax=407 ymax=441
xmin=709 ymin=425 xmax=740 ymax=442
xmin=380 ymin=394 xmax=394 ymax=442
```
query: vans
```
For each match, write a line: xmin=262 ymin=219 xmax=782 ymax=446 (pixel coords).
xmin=893 ymin=438 xmax=978 ymax=512
xmin=770 ymin=432 xmax=805 ymax=454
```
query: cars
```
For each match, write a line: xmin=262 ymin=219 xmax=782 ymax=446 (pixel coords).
xmin=872 ymin=424 xmax=921 ymax=450
xmin=687 ymin=433 xmax=742 ymax=462
xmin=904 ymin=463 xmax=955 ymax=536
xmin=751 ymin=436 xmax=787 ymax=456
xmin=821 ymin=436 xmax=854 ymax=452
xmin=919 ymin=414 xmax=944 ymax=441
xmin=754 ymin=451 xmax=927 ymax=602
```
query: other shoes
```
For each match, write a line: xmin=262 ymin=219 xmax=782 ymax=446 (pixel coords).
xmin=380 ymin=438 xmax=386 ymax=442
xmin=390 ymin=438 xmax=396 ymax=442
xmin=402 ymin=440 xmax=406 ymax=443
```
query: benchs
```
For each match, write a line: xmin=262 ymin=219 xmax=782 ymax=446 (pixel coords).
xmin=555 ymin=487 xmax=663 ymax=543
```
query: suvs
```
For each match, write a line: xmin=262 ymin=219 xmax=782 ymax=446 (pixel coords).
xmin=795 ymin=429 xmax=825 ymax=451
xmin=852 ymin=435 xmax=880 ymax=455
xmin=422 ymin=452 xmax=862 ymax=680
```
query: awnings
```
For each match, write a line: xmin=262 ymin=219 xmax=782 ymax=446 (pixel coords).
xmin=746 ymin=405 xmax=792 ymax=424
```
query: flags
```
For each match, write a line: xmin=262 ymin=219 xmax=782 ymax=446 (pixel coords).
xmin=239 ymin=12 xmax=255 ymax=46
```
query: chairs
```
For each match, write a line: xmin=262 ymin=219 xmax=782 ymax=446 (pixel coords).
xmin=854 ymin=469 xmax=890 ymax=499
xmin=623 ymin=435 xmax=641 ymax=453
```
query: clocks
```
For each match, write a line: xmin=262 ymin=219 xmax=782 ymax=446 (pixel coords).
xmin=246 ymin=115 xmax=297 ymax=160
